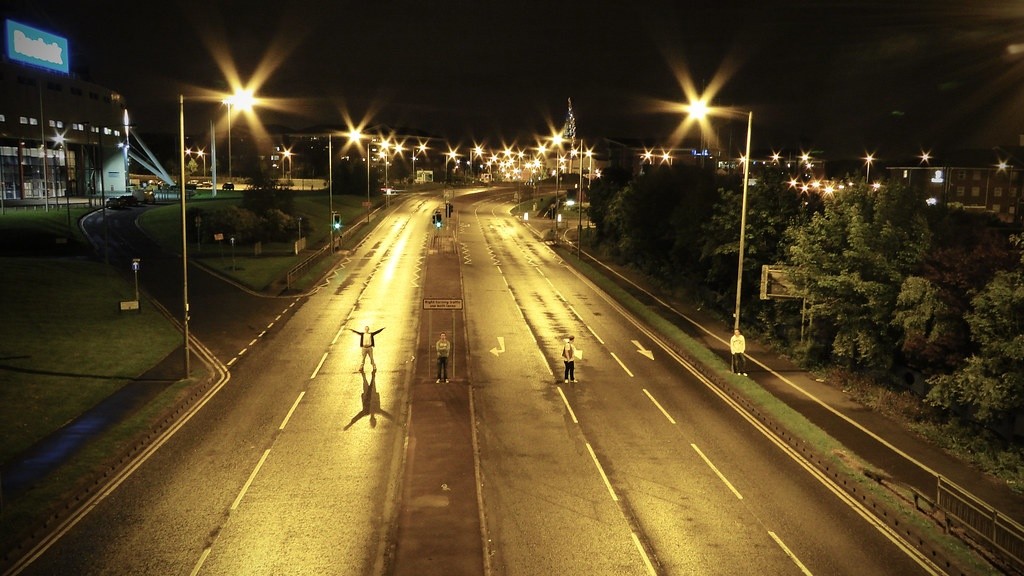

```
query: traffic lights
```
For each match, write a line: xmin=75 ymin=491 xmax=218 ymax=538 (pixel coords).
xmin=450 ymin=205 xmax=453 ymax=213
xmin=436 ymin=214 xmax=442 ymax=228
xmin=547 ymin=209 xmax=552 ymax=218
xmin=333 ymin=214 xmax=341 ymax=229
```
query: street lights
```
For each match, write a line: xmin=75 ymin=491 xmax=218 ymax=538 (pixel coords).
xmin=688 ymin=103 xmax=753 ymax=331
xmin=53 ymin=134 xmax=66 ymax=209
xmin=329 ymin=132 xmax=361 ymax=253
xmin=178 ymin=92 xmax=254 ymax=380
xmin=552 ymin=135 xmax=563 ymax=234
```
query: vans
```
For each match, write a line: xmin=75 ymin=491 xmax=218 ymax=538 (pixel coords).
xmin=188 ymin=180 xmax=212 ymax=188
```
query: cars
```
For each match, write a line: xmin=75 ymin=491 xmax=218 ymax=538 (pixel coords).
xmin=106 ymin=189 xmax=157 ymax=210
xmin=222 ymin=182 xmax=235 ymax=190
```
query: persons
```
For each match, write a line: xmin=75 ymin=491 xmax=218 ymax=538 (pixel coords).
xmin=435 ymin=332 xmax=451 ymax=383
xmin=562 ymin=335 xmax=578 ymax=384
xmin=348 ymin=326 xmax=386 ymax=372
xmin=730 ymin=328 xmax=748 ymax=377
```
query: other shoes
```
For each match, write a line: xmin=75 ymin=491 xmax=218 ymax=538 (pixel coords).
xmin=743 ymin=373 xmax=747 ymax=376
xmin=738 ymin=373 xmax=740 ymax=376
xmin=564 ymin=379 xmax=568 ymax=384
xmin=571 ymin=379 xmax=578 ymax=382
xmin=372 ymin=369 xmax=376 ymax=373
xmin=445 ymin=379 xmax=449 ymax=383
xmin=359 ymin=368 xmax=363 ymax=371
xmin=436 ymin=378 xmax=441 ymax=384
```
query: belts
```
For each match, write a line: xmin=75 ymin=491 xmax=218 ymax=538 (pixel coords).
xmin=363 ymin=345 xmax=373 ymax=348
